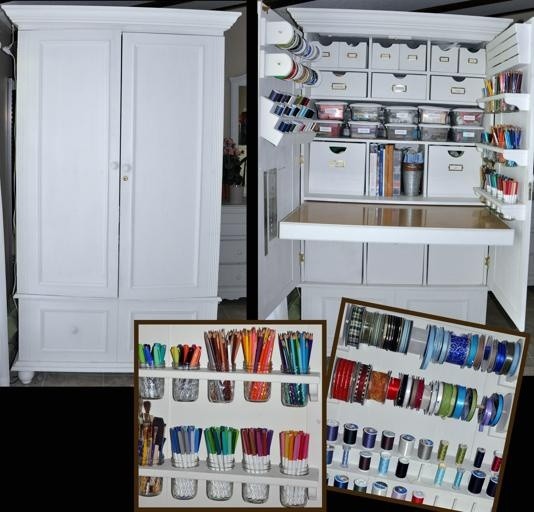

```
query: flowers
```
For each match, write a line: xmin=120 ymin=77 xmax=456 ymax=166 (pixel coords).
xmin=224 ymin=136 xmax=247 ymax=184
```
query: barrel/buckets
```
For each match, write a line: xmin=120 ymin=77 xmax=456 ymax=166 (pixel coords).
xmin=402 ymin=162 xmax=421 ymax=197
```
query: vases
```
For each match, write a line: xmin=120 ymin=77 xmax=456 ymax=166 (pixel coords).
xmin=230 ymin=184 xmax=243 ymax=204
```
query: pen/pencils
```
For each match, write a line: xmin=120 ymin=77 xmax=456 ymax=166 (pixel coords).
xmin=483 ymin=70 xmax=523 ymax=113
xmin=137 ymin=327 xmax=313 ymax=407
xmin=170 ymin=426 xmax=309 ymax=506
xmin=481 ymin=164 xmax=518 ymax=194
xmin=491 ymin=124 xmax=520 ymax=149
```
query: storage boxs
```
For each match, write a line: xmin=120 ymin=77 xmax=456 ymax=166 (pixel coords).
xmin=308 ymin=37 xmax=487 ymax=102
xmin=310 ymin=100 xmax=485 ymax=143
xmin=308 ymin=140 xmax=366 ymax=197
xmin=427 ymin=145 xmax=482 ymax=198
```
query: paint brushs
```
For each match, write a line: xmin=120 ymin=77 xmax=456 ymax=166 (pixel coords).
xmin=137 ymin=401 xmax=166 ymax=496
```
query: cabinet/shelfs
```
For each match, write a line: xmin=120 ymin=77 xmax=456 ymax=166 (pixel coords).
xmin=131 ymin=318 xmax=329 ymax=511
xmin=258 ymin=0 xmax=534 ymax=332
xmin=0 ymin=1 xmax=242 ymax=384
xmin=218 ymin=204 xmax=247 ymax=302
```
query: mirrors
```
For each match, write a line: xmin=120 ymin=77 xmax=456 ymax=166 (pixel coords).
xmin=228 ymin=73 xmax=247 ymax=197
